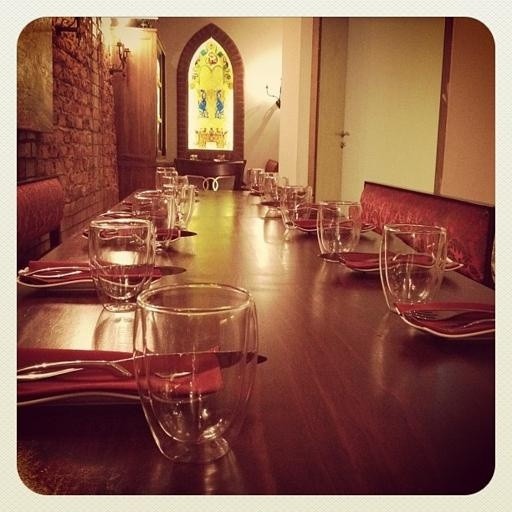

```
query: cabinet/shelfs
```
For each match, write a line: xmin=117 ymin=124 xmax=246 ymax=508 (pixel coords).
xmin=106 ymin=28 xmax=167 ymax=201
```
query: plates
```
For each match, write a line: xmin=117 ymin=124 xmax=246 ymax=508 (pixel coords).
xmin=315 ymin=249 xmax=463 ymax=277
xmin=282 ymin=218 xmax=377 ymax=236
xmin=261 ymin=201 xmax=340 ymax=214
xmin=81 ymin=226 xmax=183 ymax=246
xmin=17 ymin=390 xmax=214 ymax=406
xmin=391 ymin=300 xmax=494 ymax=342
xmin=18 ymin=278 xmax=160 ymax=296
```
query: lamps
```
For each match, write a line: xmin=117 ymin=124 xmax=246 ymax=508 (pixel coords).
xmin=109 ymin=26 xmax=142 ymax=76
xmin=262 ymin=79 xmax=281 ymax=109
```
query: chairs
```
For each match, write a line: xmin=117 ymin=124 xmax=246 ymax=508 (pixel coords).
xmin=174 ymin=157 xmax=246 ymax=191
xmin=353 ymin=179 xmax=494 ymax=288
xmin=16 ymin=174 xmax=65 ymax=269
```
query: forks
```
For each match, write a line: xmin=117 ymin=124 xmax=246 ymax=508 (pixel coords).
xmin=410 ymin=307 xmax=494 ymax=321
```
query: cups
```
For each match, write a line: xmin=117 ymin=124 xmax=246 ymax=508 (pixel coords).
xmin=378 ymin=224 xmax=447 ymax=311
xmin=88 ymin=216 xmax=156 ymax=313
xmin=133 ymin=285 xmax=260 ymax=466
xmin=93 ymin=304 xmax=159 ymax=355
xmin=246 ymin=165 xmax=311 ymax=230
xmin=131 ymin=166 xmax=199 ymax=254
xmin=317 ymin=200 xmax=364 ymax=262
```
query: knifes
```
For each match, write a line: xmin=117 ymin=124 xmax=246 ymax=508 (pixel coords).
xmin=23 ymin=266 xmax=186 ymax=282
xmin=18 ymin=348 xmax=267 ymax=382
